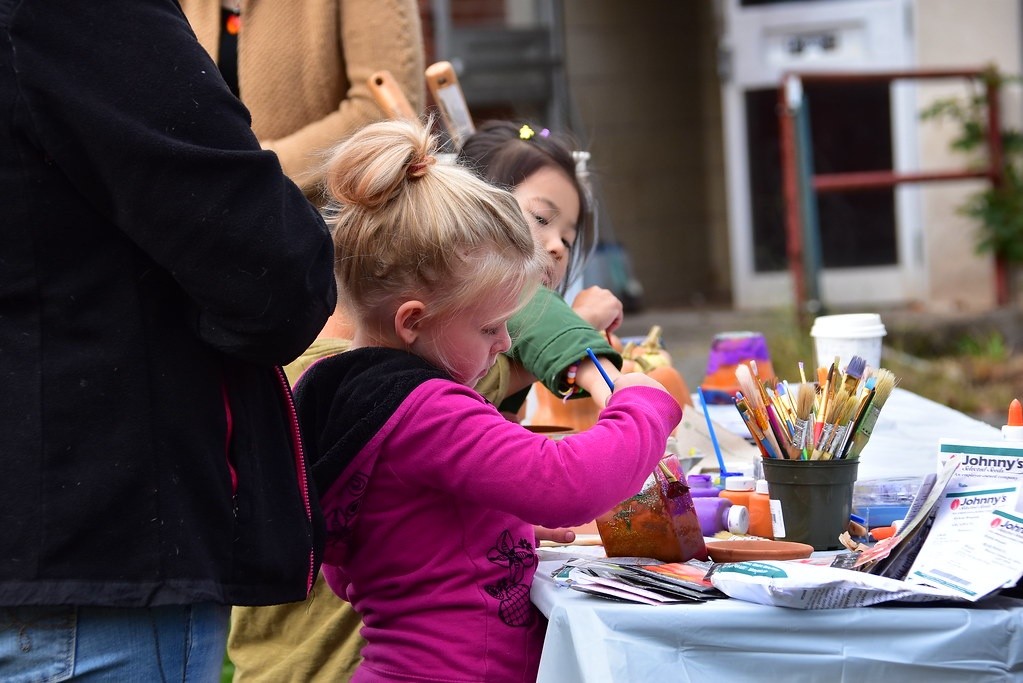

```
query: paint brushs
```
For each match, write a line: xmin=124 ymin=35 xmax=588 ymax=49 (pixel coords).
xmin=585 ymin=349 xmax=690 ymax=497
xmin=810 ymin=354 xmax=867 ymax=459
xmin=790 ymin=362 xmax=833 ymax=461
xmin=833 ymin=368 xmax=902 ymax=459
xmin=697 ymin=386 xmax=727 ymax=473
xmin=729 ymin=360 xmax=798 ymax=461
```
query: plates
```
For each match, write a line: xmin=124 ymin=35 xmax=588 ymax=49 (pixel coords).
xmin=705 ymin=541 xmax=813 ymax=562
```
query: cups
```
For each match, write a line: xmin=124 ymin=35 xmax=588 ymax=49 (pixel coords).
xmin=760 ymin=456 xmax=859 ymax=551
xmin=809 ymin=313 xmax=888 ymax=374
xmin=700 ymin=332 xmax=778 ymax=406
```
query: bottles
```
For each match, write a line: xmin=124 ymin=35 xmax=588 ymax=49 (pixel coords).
xmin=686 ymin=475 xmax=774 ymax=541
xmin=1002 ymin=398 xmax=1023 ymax=444
xmin=870 ymin=520 xmax=905 ymax=542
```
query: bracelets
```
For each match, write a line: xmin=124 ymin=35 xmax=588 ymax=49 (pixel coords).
xmin=558 ymin=357 xmax=584 ymax=404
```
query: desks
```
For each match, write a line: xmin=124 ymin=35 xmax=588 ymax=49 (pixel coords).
xmin=529 ymin=378 xmax=1023 ymax=682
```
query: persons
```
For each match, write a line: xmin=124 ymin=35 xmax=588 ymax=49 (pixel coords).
xmin=0 ymin=0 xmax=684 ymax=683
